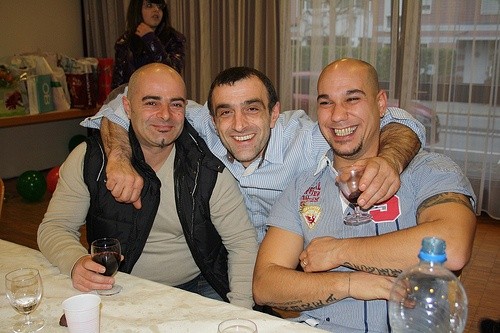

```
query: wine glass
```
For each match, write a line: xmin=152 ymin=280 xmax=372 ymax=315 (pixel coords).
xmin=91 ymin=238 xmax=122 ymax=296
xmin=4 ymin=268 xmax=47 ymax=333
xmin=338 ymin=165 xmax=373 ymax=224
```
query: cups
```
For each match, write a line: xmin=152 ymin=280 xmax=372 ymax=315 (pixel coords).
xmin=61 ymin=294 xmax=101 ymax=333
xmin=218 ymin=318 xmax=258 ymax=333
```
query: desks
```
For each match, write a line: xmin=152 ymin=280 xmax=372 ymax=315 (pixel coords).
xmin=0 ymin=107 xmax=98 ymax=179
xmin=0 ymin=239 xmax=331 ymax=333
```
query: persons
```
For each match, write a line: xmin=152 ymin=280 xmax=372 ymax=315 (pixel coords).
xmin=36 ymin=62 xmax=258 ymax=310
xmin=251 ymin=59 xmax=477 ymax=333
xmin=78 ymin=67 xmax=425 ymax=243
xmin=110 ymin=0 xmax=186 ymax=90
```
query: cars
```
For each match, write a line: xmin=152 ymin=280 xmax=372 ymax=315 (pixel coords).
xmin=291 ymin=71 xmax=442 ymax=143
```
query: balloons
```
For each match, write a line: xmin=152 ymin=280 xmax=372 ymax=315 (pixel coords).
xmin=17 ymin=171 xmax=47 ymax=203
xmin=46 ymin=167 xmax=60 ymax=193
xmin=69 ymin=134 xmax=86 ymax=152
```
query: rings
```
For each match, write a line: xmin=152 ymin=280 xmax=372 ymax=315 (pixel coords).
xmin=302 ymin=258 xmax=308 ymax=266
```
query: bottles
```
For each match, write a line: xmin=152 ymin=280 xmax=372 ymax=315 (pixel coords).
xmin=388 ymin=236 xmax=468 ymax=333
